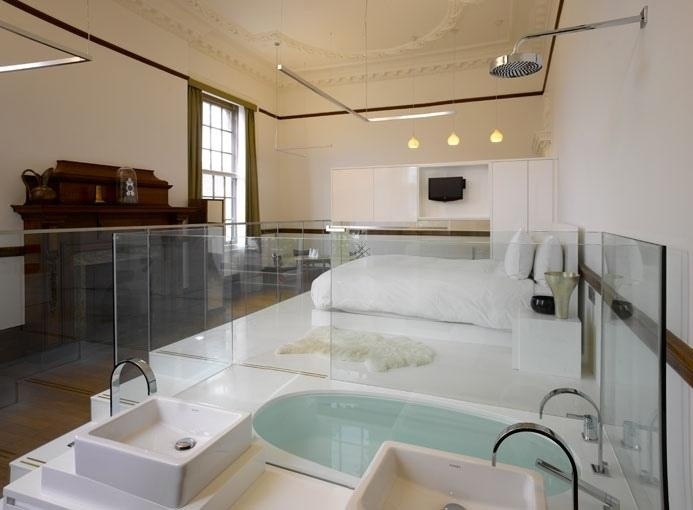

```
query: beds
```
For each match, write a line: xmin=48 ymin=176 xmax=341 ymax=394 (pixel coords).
xmin=309 ymin=221 xmax=579 ymax=348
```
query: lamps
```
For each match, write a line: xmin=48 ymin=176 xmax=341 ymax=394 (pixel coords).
xmin=407 ymin=19 xmax=504 ymax=149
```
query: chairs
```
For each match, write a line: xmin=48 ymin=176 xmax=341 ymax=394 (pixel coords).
xmin=271 ymin=249 xmax=331 ymax=303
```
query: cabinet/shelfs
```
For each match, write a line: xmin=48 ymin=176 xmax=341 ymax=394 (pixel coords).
xmin=187 ymin=198 xmax=225 ymax=319
xmin=489 ymin=160 xmax=557 ymax=259
xmin=329 ymin=163 xmax=420 ymax=223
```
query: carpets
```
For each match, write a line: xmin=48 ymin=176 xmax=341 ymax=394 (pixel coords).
xmin=277 ymin=325 xmax=435 ymax=373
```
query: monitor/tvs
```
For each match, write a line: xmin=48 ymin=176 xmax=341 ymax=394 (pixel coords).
xmin=429 ymin=177 xmax=462 ymax=201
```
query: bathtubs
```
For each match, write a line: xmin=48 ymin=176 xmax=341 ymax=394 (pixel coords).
xmin=253 ymin=374 xmax=582 ymax=499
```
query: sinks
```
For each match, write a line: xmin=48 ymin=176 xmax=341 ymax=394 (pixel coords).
xmin=74 ymin=395 xmax=253 ymax=509
xmin=341 ymin=441 xmax=549 ymax=509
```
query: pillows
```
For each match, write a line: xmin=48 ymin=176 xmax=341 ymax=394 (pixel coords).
xmin=504 ymin=228 xmax=536 ymax=280
xmin=531 ymin=234 xmax=564 ymax=286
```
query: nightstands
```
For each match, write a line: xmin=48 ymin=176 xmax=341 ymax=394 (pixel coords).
xmin=515 ymin=307 xmax=582 ymax=380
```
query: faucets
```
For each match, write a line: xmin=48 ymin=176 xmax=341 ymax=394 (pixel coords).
xmin=636 ymin=410 xmax=661 ymax=486
xmin=534 ymin=456 xmax=621 ymax=510
xmin=108 ymin=357 xmax=158 ymax=417
xmin=539 ymin=386 xmax=608 ymax=474
xmin=491 ymin=421 xmax=579 ymax=510
